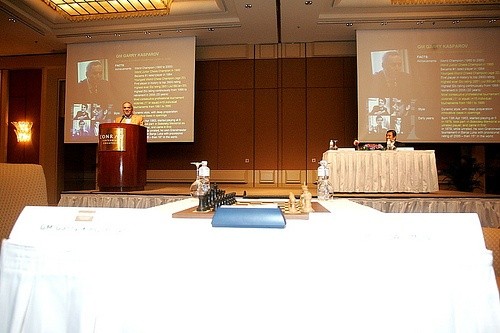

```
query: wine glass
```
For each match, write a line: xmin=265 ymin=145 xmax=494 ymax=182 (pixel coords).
xmin=333 ymin=140 xmax=338 ymax=150
xmin=317 ymin=160 xmax=334 ymax=201
xmin=190 ymin=162 xmax=208 ymax=197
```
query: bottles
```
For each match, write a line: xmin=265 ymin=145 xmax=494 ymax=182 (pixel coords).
xmin=330 ymin=140 xmax=333 ymax=149
xmin=199 ymin=161 xmax=210 ymax=191
xmin=318 ymin=161 xmax=329 ymax=179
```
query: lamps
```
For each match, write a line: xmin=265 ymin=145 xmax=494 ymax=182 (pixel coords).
xmin=11 ymin=120 xmax=34 ymax=143
xmin=41 ymin=0 xmax=174 ymax=23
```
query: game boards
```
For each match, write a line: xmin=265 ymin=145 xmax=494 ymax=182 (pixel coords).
xmin=172 ymin=202 xmax=332 ymax=218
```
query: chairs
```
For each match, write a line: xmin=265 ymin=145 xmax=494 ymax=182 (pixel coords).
xmin=0 ymin=163 xmax=48 ymax=248
xmin=481 ymin=227 xmax=500 ymax=289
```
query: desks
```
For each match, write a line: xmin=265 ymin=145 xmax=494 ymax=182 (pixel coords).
xmin=0 ymin=196 xmax=500 ymax=333
xmin=322 ymin=150 xmax=440 ymax=194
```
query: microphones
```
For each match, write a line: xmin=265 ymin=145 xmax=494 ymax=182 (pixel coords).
xmin=119 ymin=114 xmax=126 ymax=123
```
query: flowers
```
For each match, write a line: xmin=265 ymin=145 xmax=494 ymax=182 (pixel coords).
xmin=353 ymin=140 xmax=359 ymax=145
xmin=363 ymin=144 xmax=383 ymax=150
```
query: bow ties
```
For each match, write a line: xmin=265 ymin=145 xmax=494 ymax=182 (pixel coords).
xmin=125 ymin=115 xmax=132 ymax=119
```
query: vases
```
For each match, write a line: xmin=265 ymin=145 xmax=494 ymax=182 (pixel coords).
xmin=356 ymin=145 xmax=359 ymax=150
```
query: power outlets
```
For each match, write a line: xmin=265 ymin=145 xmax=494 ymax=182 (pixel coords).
xmin=312 ymin=158 xmax=317 ymax=163
xmin=244 ymin=158 xmax=250 ymax=164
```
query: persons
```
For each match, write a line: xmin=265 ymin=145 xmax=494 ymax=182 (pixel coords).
xmin=372 ymin=51 xmax=411 ymax=96
xmin=383 ymin=130 xmax=404 ymax=151
xmin=368 ymin=116 xmax=388 ymax=135
xmin=369 ymin=98 xmax=390 ymax=116
xmin=391 ymin=97 xmax=415 ymax=117
xmin=74 ymin=104 xmax=90 ymax=119
xmin=73 ymin=120 xmax=89 ymax=136
xmin=77 ymin=60 xmax=110 ymax=103
xmin=115 ymin=102 xmax=143 ymax=126
xmin=390 ymin=117 xmax=409 ymax=140
xmin=91 ymin=103 xmax=113 ymax=120
